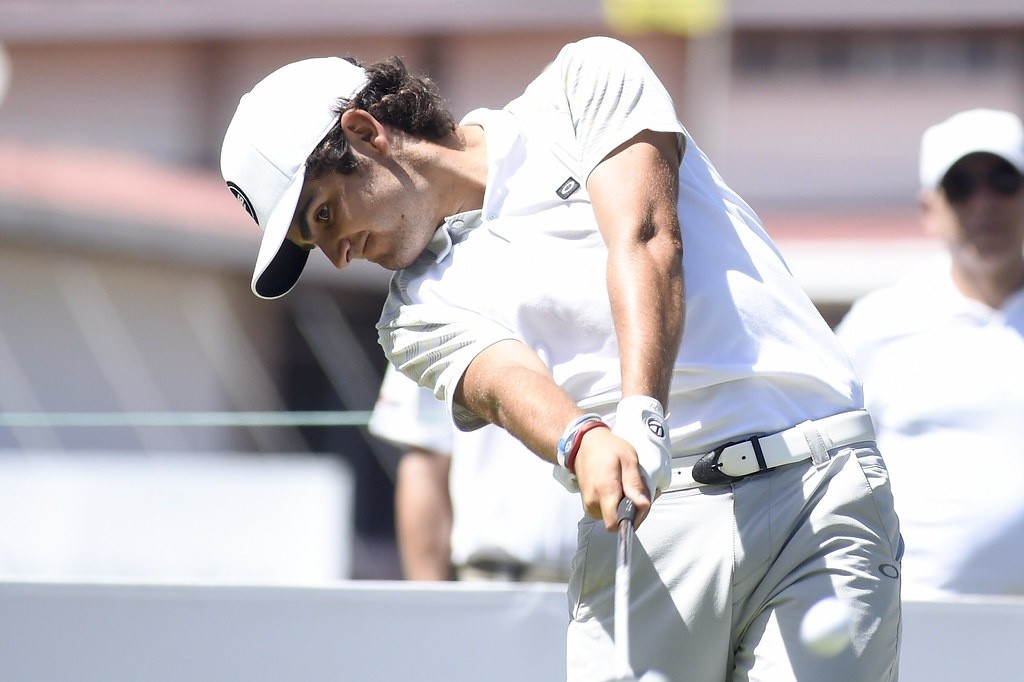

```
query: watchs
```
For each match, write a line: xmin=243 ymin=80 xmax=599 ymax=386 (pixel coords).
xmin=560 ymin=419 xmax=610 ymax=477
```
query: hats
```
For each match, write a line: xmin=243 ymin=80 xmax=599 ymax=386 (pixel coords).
xmin=220 ymin=56 xmax=372 ymax=299
xmin=920 ymin=104 xmax=1024 ymax=190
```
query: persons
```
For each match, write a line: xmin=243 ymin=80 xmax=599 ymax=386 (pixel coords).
xmin=832 ymin=106 xmax=1024 ymax=601
xmin=368 ymin=357 xmax=585 ymax=587
xmin=214 ymin=35 xmax=904 ymax=681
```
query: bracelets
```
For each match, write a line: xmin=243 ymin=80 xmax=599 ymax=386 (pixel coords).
xmin=555 ymin=413 xmax=602 ymax=471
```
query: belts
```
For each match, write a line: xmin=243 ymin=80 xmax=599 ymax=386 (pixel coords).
xmin=653 ymin=408 xmax=875 ymax=493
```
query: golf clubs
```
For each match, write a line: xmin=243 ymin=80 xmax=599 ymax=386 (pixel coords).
xmin=604 ymin=497 xmax=637 ymax=682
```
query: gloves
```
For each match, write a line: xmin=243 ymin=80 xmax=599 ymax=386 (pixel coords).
xmin=610 ymin=395 xmax=673 ymax=504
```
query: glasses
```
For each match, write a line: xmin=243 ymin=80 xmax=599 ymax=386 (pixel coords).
xmin=941 ymin=166 xmax=1021 ymax=204
